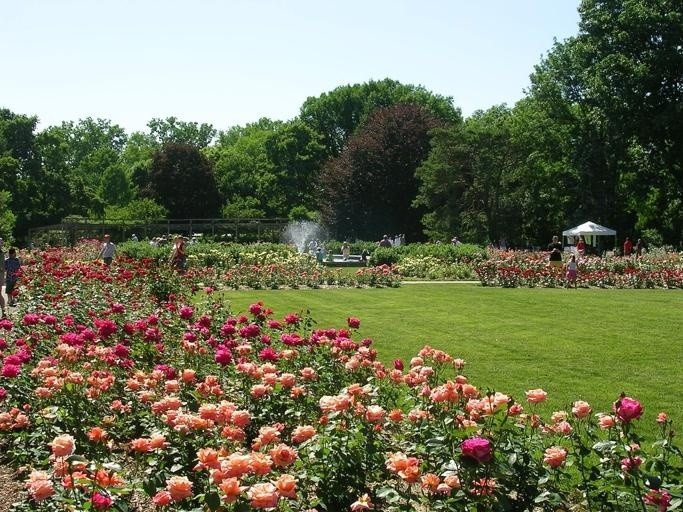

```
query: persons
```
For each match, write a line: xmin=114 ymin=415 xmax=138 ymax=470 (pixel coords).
xmin=96 ymin=235 xmax=119 ymax=265
xmin=4 ymin=248 xmax=20 ymax=307
xmin=451 ymin=239 xmax=460 ymax=246
xmin=547 ymin=235 xmax=642 ymax=289
xmin=0 ymin=238 xmax=7 ymax=321
xmin=289 ymin=234 xmax=392 ymax=265
xmin=453 ymin=237 xmax=459 ymax=246
xmin=131 ymin=234 xmax=198 ymax=273
